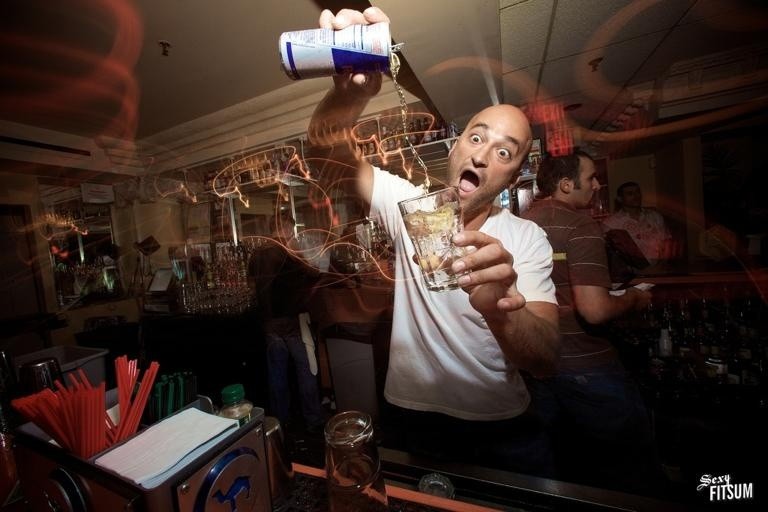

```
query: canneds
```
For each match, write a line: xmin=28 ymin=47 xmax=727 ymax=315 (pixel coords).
xmin=277 ymin=21 xmax=405 ymax=81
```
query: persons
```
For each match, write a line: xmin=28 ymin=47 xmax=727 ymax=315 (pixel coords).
xmin=245 ymin=210 xmax=362 ymax=447
xmin=303 ymin=6 xmax=563 ymax=449
xmin=601 ymin=182 xmax=671 ymax=275
xmin=516 ymin=149 xmax=657 ymax=441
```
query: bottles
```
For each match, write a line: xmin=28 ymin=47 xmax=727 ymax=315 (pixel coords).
xmin=322 ymin=409 xmax=392 ymax=512
xmin=220 ymin=383 xmax=254 ymax=425
xmin=613 ymin=293 xmax=768 ymax=444
xmin=210 ymin=240 xmax=271 ymax=286
xmin=200 ymin=168 xmax=233 ymax=242
xmin=358 ymin=114 xmax=447 ymax=156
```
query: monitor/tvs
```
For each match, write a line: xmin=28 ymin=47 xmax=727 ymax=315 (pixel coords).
xmin=519 ymin=138 xmax=542 ymax=181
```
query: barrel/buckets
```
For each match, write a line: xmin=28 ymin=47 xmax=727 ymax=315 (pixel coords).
xmin=10 ymin=342 xmax=108 ymax=386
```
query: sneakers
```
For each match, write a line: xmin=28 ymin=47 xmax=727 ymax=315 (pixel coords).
xmin=288 ymin=436 xmax=308 ymax=455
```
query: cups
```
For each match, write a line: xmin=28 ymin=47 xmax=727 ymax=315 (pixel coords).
xmin=397 ymin=186 xmax=475 ymax=293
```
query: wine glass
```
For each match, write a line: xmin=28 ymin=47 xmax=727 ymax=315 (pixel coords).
xmin=248 ymin=152 xmax=281 ymax=187
xmin=180 ymin=282 xmax=255 ymax=315
